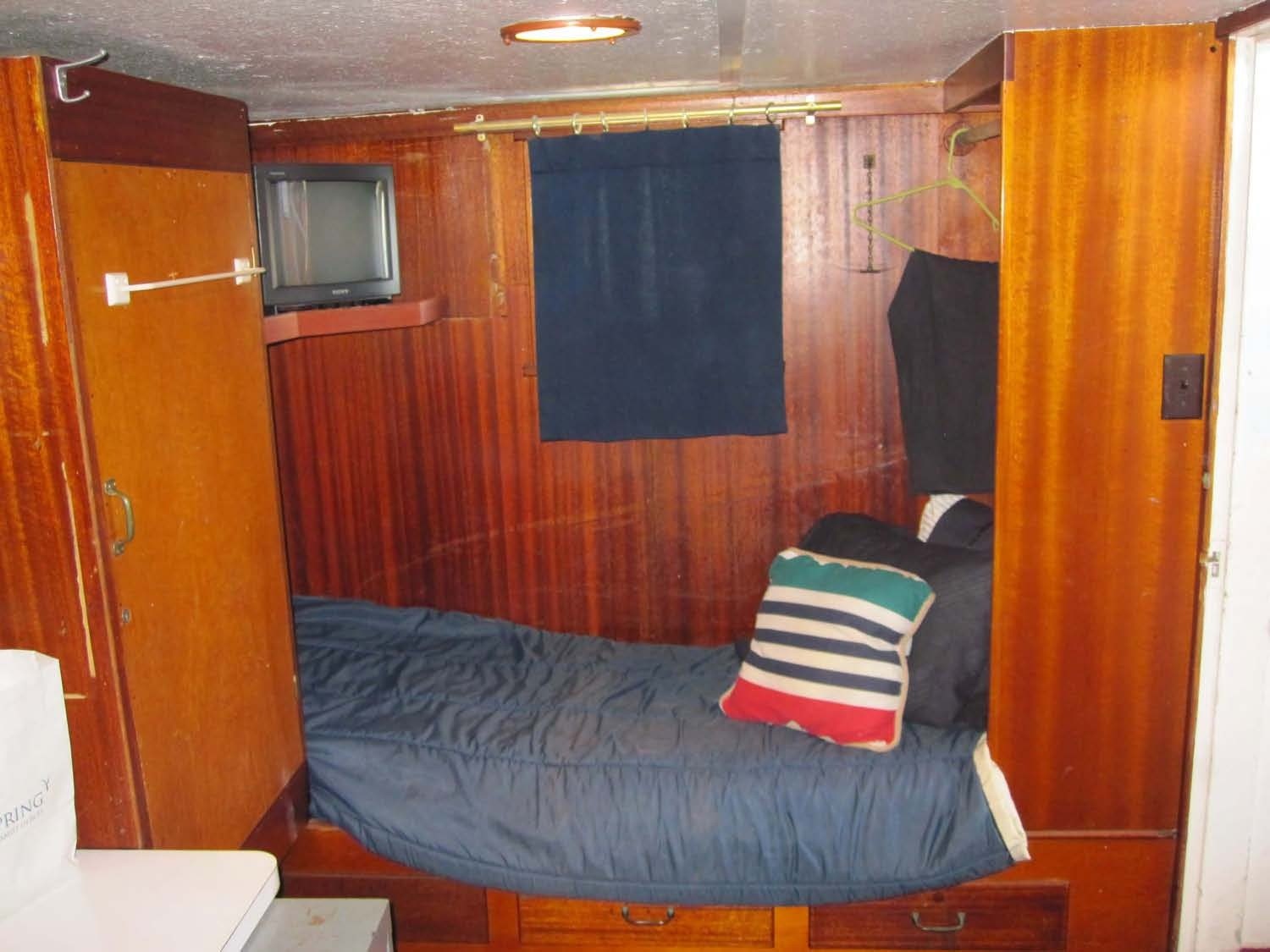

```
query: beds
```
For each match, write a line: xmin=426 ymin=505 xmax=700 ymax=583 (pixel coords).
xmin=291 ymin=598 xmax=1073 ymax=952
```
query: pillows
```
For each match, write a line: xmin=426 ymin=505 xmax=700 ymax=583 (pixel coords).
xmin=918 ymin=492 xmax=994 ymax=553
xmin=718 ymin=546 xmax=935 ymax=752
xmin=797 ymin=514 xmax=996 ymax=726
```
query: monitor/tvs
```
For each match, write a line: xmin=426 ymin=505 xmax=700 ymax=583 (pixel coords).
xmin=250 ymin=163 xmax=402 ymax=316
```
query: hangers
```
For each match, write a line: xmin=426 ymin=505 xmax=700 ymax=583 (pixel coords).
xmin=850 ymin=130 xmax=999 ymax=262
